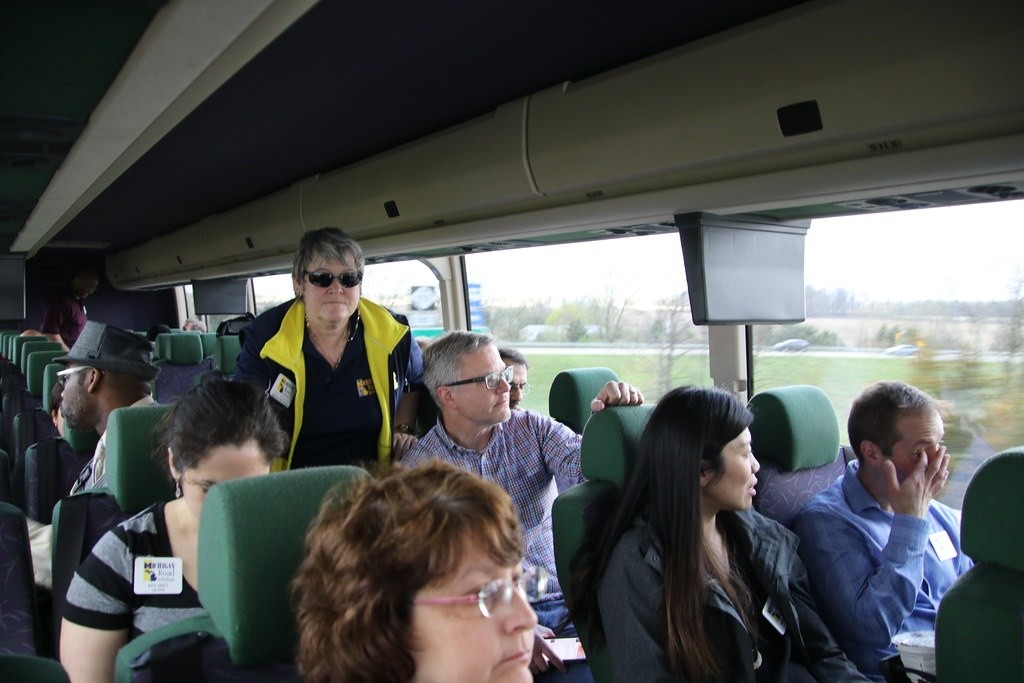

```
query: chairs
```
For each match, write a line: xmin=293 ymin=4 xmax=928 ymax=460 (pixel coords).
xmin=0 ymin=330 xmax=1023 ymax=683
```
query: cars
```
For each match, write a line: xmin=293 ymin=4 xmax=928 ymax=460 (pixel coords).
xmin=773 ymin=339 xmax=810 ymax=352
xmin=887 ymin=342 xmax=920 ymax=356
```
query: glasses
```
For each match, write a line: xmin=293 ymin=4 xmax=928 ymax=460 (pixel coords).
xmin=302 ymin=268 xmax=361 ymax=289
xmin=439 ymin=365 xmax=513 ymax=390
xmin=403 ymin=565 xmax=551 ymax=618
xmin=56 ymin=366 xmax=106 ymax=387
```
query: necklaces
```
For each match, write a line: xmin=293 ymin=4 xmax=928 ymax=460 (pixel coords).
xmin=305 ymin=316 xmax=349 ymax=367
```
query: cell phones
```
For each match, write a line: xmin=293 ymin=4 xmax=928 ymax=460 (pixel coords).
xmin=541 ymin=638 xmax=586 ymax=664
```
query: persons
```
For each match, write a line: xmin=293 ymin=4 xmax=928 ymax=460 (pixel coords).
xmin=60 ymin=382 xmax=283 ymax=683
xmin=587 ymin=384 xmax=875 ymax=683
xmin=796 ymin=381 xmax=974 ymax=683
xmin=401 ymin=332 xmax=644 ymax=683
xmin=498 ymin=348 xmax=529 ymax=411
xmin=298 ymin=463 xmax=538 ymax=683
xmin=234 ymin=228 xmax=438 ymax=470
xmin=16 ymin=269 xmax=208 ymax=587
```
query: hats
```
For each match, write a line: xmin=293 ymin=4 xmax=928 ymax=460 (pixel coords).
xmin=50 ymin=319 xmax=161 ymax=380
